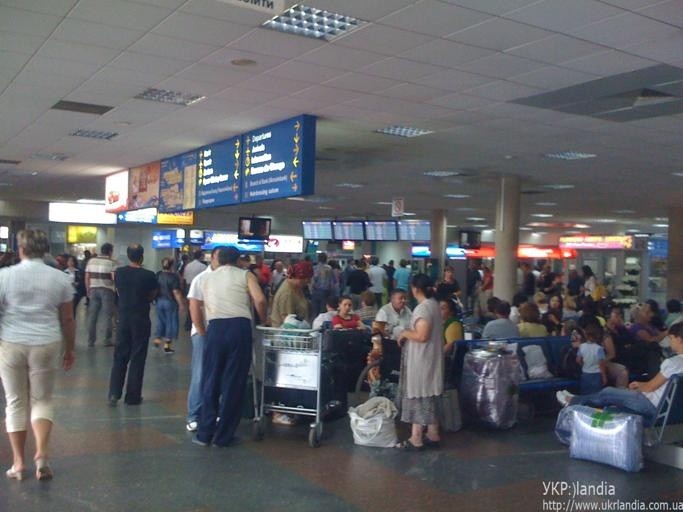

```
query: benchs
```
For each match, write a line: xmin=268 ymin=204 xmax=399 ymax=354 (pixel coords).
xmin=441 ymin=339 xmax=470 ymax=397
xmin=462 ymin=335 xmax=582 ymax=417
xmin=603 ymin=373 xmax=682 ymax=475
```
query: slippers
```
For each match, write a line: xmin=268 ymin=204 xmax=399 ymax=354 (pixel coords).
xmin=423 ymin=434 xmax=440 ymax=448
xmin=6 ymin=464 xmax=25 ymax=479
xmin=34 ymin=455 xmax=53 ymax=481
xmin=395 ymin=440 xmax=423 ymax=451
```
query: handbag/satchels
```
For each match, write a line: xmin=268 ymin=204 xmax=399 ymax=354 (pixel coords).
xmin=438 ymin=387 xmax=463 ymax=432
xmin=569 ymin=406 xmax=643 ymax=472
xmin=346 ymin=396 xmax=398 ymax=446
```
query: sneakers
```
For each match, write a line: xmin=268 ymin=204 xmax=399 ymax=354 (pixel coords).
xmin=563 ymin=390 xmax=575 ymax=398
xmin=186 ymin=421 xmax=199 ymax=432
xmin=556 ymin=390 xmax=569 ymax=408
xmin=164 ymin=348 xmax=176 ymax=353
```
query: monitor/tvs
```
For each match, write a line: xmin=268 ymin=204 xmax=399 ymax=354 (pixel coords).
xmin=364 ymin=220 xmax=397 ymax=241
xmin=459 ymin=230 xmax=481 ymax=249
xmin=302 ymin=220 xmax=333 ymax=241
xmin=397 ymin=219 xmax=432 ymax=241
xmin=238 ymin=217 xmax=271 ymax=240
xmin=333 ymin=220 xmax=364 ymax=240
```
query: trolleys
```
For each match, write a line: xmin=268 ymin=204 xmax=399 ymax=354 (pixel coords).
xmin=251 ymin=325 xmax=343 ymax=448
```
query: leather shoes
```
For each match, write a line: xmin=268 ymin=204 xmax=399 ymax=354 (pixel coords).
xmin=106 ymin=396 xmax=117 ymax=406
xmin=192 ymin=434 xmax=206 ymax=446
xmin=213 ymin=436 xmax=241 ymax=448
xmin=125 ymin=397 xmax=144 ymax=404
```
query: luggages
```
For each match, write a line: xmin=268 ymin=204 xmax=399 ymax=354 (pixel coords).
xmin=458 ymin=347 xmax=520 ymax=430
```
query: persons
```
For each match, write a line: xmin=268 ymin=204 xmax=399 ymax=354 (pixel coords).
xmin=373 ymin=288 xmax=411 ymax=342
xmin=394 ymin=274 xmax=445 ymax=451
xmin=520 ymin=264 xmax=536 ymax=302
xmin=1 ymin=229 xmax=77 ymax=481
xmin=85 ymin=243 xmax=117 ymax=348
xmin=604 ymin=305 xmax=645 ymax=370
xmin=82 ymin=250 xmax=93 ymax=304
xmin=541 ymin=294 xmax=563 ymax=329
xmin=271 ymin=262 xmax=287 ymax=296
xmin=240 ymin=254 xmax=261 ymax=283
xmin=328 ymin=259 xmax=343 ymax=297
xmin=312 ymin=298 xmax=337 ymax=331
xmin=537 ymin=259 xmax=562 ymax=291
xmin=576 ymin=323 xmax=607 ymax=390
xmin=187 ymin=246 xmax=224 ymax=432
xmin=270 ymin=260 xmax=314 ymax=328
xmin=478 ymin=296 xmax=500 ymax=325
xmin=510 ymin=293 xmax=527 ymax=322
xmin=631 ymin=303 xmax=669 ymax=341
xmin=132 ymin=177 xmax=139 ymax=200
xmin=439 ymin=297 xmax=463 ymax=355
xmin=394 ymin=259 xmax=413 ymax=291
xmin=532 ymin=292 xmax=548 ymax=312
xmin=192 ymin=246 xmax=267 ymax=447
xmin=516 ymin=263 xmax=524 ymax=290
xmin=345 ymin=261 xmax=372 ymax=299
xmin=580 ymin=265 xmax=599 ymax=296
xmin=571 ymin=311 xmax=628 ymax=386
xmin=473 ymin=267 xmax=493 ymax=299
xmin=332 ymin=295 xmax=365 ymax=330
xmin=563 ymin=296 xmax=579 ymax=322
xmin=557 ymin=323 xmax=683 ymax=415
xmin=154 ymin=257 xmax=185 ymax=354
xmin=107 ymin=243 xmax=158 ymax=406
xmin=367 ymin=255 xmax=387 ymax=308
xmin=480 ymin=300 xmax=519 ymax=338
xmin=308 ymin=253 xmax=337 ymax=315
xmin=183 ymin=251 xmax=207 ymax=336
xmin=465 ymin=262 xmax=481 ymax=314
xmin=518 ymin=303 xmax=548 ymax=337
xmin=255 ymin=254 xmax=270 ymax=318
xmin=64 ymin=258 xmax=82 ymax=316
xmin=437 ymin=266 xmax=462 ymax=296
xmin=344 ymin=258 xmax=355 ymax=277
xmin=568 ymin=270 xmax=581 ymax=297
